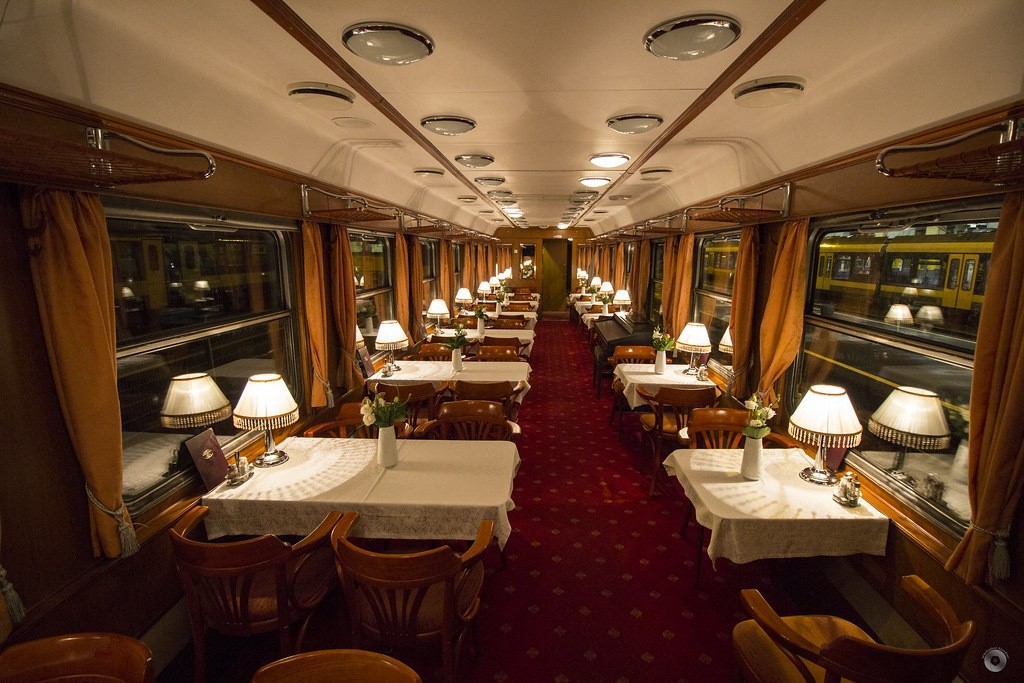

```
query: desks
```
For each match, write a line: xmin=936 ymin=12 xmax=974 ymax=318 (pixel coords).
xmin=569 ymin=290 xmax=888 ymax=600
xmin=196 ymin=287 xmax=546 ymax=556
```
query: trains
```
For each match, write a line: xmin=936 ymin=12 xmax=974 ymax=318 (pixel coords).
xmin=691 ymin=222 xmax=997 ymax=321
xmin=104 ymin=230 xmax=391 ymax=331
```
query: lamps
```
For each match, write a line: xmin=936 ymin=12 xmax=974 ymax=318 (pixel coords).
xmin=882 ymin=303 xmax=915 ymax=333
xmin=158 ymin=368 xmax=230 ymax=432
xmin=914 ymin=304 xmax=945 ymax=335
xmin=559 ymin=14 xmax=809 ymax=232
xmin=600 ymin=281 xmax=615 ymax=302
xmin=867 ymin=383 xmax=953 ymax=491
xmin=590 ymin=277 xmax=602 ymax=296
xmin=284 ymin=18 xmax=527 ymax=228
xmin=474 ymin=281 xmax=492 ymax=303
xmin=489 ymin=275 xmax=498 ymax=293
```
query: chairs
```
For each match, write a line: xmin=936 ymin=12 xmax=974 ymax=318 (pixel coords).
xmin=0 ymin=629 xmax=156 ymax=683
xmin=327 ymin=510 xmax=495 ymax=683
xmin=173 ymin=504 xmax=342 ymax=680
xmin=731 ymin=576 xmax=981 ymax=683
xmin=244 ymin=647 xmax=425 ymax=683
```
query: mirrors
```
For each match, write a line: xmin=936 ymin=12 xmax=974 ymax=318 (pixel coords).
xmin=519 ymin=242 xmax=538 ymax=281
